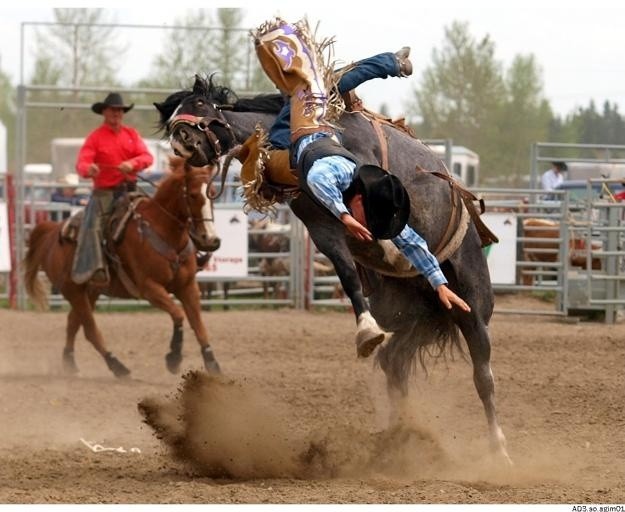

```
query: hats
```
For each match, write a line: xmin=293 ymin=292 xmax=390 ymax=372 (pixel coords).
xmin=91 ymin=92 xmax=133 ymax=115
xmin=358 ymin=163 xmax=411 ymax=240
xmin=553 ymin=162 xmax=568 ymax=171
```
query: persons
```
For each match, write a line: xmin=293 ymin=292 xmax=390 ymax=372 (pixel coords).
xmin=263 ymin=43 xmax=474 ymax=312
xmin=537 ymin=161 xmax=569 ymax=213
xmin=71 ymin=91 xmax=153 ymax=289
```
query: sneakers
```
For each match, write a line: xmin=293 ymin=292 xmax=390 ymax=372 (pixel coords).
xmin=395 ymin=46 xmax=413 ymax=77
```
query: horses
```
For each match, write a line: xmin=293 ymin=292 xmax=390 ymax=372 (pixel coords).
xmin=151 ymin=71 xmax=515 ymax=483
xmin=522 ymin=218 xmax=603 ymax=280
xmin=19 ymin=154 xmax=222 ymax=383
